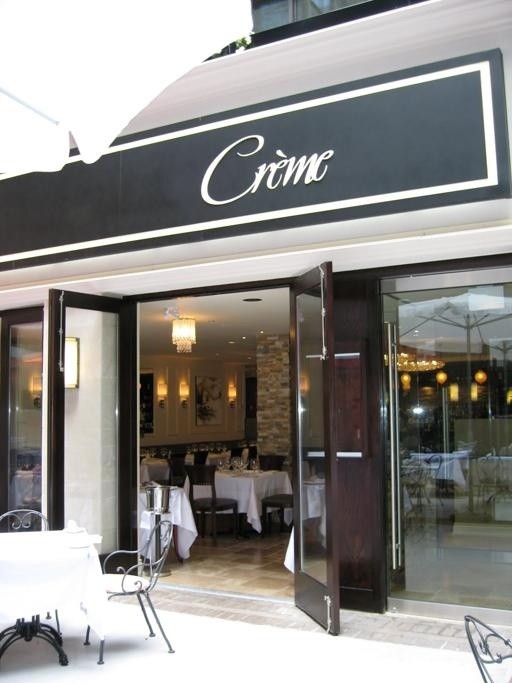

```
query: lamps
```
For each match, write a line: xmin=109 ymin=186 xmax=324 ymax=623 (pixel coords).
xmin=385 ymin=338 xmax=489 ymax=401
xmin=225 ymin=375 xmax=240 ymax=410
xmin=64 ymin=336 xmax=83 ymax=391
xmin=156 ymin=371 xmax=190 ymax=411
xmin=28 ymin=370 xmax=43 ymax=411
xmin=169 ymin=317 xmax=200 ymax=356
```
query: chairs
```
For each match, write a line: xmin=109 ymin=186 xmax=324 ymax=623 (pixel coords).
xmin=1 ymin=508 xmax=64 ymax=637
xmin=184 ymin=463 xmax=239 ymax=549
xmin=464 ymin=614 xmax=512 ymax=683
xmin=138 ymin=444 xmax=295 ymax=515
xmin=261 ymin=493 xmax=295 ymax=539
xmin=83 ymin=520 xmax=176 ymax=665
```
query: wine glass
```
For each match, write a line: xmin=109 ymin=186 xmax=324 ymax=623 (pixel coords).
xmin=216 ymin=457 xmax=258 ymax=474
xmin=141 ymin=448 xmax=167 ymax=459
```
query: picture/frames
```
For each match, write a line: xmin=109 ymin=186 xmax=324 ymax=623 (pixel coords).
xmin=194 ymin=371 xmax=227 ymax=427
xmin=139 ymin=365 xmax=156 ymax=435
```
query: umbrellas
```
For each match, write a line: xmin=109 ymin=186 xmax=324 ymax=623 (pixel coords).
xmin=0 ymin=0 xmax=256 ymax=181
